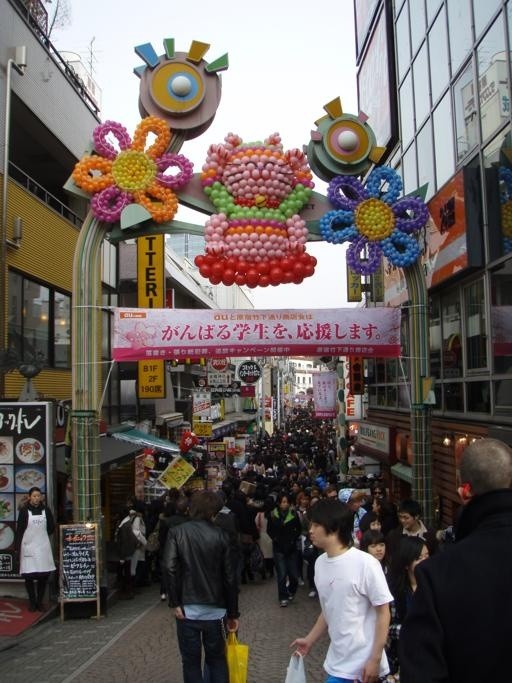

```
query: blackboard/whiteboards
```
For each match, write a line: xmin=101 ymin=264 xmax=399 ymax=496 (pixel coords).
xmin=59 ymin=523 xmax=100 ymax=602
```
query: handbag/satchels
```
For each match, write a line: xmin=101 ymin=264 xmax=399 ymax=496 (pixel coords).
xmin=225 ymin=631 xmax=249 ymax=683
xmin=241 ymin=534 xmax=253 ymax=545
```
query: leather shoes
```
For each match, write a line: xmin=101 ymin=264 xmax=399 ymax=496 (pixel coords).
xmin=161 ymin=593 xmax=166 ymax=600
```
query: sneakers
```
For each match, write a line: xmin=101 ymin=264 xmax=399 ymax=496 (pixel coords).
xmin=279 ymin=593 xmax=294 ymax=607
xmin=308 ymin=592 xmax=317 ymax=598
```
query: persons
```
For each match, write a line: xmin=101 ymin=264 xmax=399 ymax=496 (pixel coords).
xmin=287 ymin=494 xmax=399 ymax=683
xmin=384 ymin=535 xmax=432 ymax=681
xmin=395 ymin=435 xmax=511 ymax=681
xmin=8 ymin=485 xmax=57 ymax=614
xmin=159 ymin=486 xmax=241 ymax=681
xmin=106 ymin=396 xmax=455 ymax=608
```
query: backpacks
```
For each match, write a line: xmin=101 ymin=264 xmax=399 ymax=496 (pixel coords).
xmin=117 ymin=514 xmax=139 ymax=559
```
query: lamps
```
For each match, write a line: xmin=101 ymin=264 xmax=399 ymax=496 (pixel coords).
xmin=442 ymin=433 xmax=451 ymax=447
xmin=457 ymin=434 xmax=468 ymax=445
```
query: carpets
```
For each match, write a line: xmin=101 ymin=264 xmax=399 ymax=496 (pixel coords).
xmin=1 ymin=597 xmax=40 ymax=636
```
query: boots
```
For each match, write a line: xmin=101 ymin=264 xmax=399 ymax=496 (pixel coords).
xmin=26 ymin=583 xmax=46 ymax=611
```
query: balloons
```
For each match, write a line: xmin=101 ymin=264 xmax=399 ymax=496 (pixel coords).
xmin=71 ymin=113 xmax=195 ymax=225
xmin=316 ymin=163 xmax=431 ymax=278
xmin=194 ymin=126 xmax=318 ymax=287
